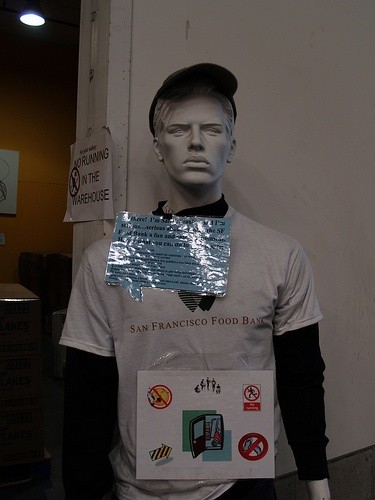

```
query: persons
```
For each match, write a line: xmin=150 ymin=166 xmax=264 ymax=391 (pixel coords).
xmin=58 ymin=62 xmax=332 ymax=500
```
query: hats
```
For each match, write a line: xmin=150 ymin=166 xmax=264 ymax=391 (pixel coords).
xmin=148 ymin=62 xmax=238 ymax=138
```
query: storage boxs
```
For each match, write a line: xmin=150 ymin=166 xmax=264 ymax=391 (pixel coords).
xmin=0 ymin=283 xmax=40 ymax=353
xmin=0 ymin=408 xmax=45 ymax=460
xmin=0 ymin=354 xmax=44 ymax=410
